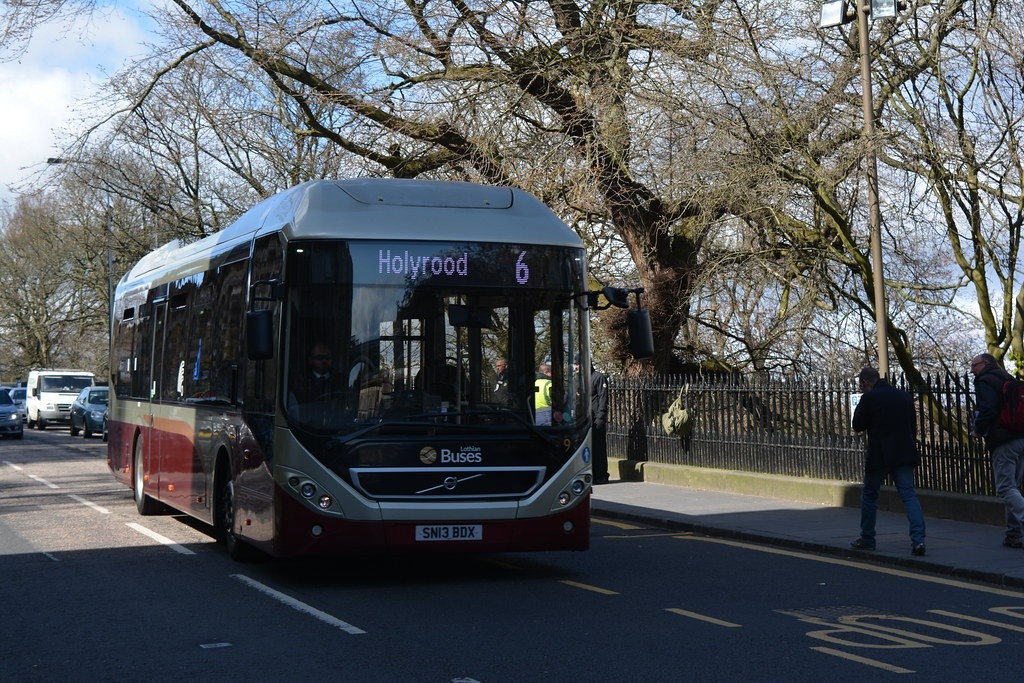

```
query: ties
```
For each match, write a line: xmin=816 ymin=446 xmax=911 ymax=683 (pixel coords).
xmin=320 ymin=375 xmax=326 ymax=382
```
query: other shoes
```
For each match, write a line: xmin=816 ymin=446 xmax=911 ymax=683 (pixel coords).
xmin=912 ymin=543 xmax=926 ymax=555
xmin=1003 ymin=536 xmax=1022 ymax=547
xmin=592 ymin=478 xmax=608 ymax=484
xmin=851 ymin=537 xmax=875 ymax=550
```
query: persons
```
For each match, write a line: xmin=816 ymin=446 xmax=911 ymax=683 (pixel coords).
xmin=492 ymin=357 xmax=509 ymax=411
xmin=534 ymin=363 xmax=552 ymax=426
xmin=969 ymin=353 xmax=1023 ymax=546
xmin=572 ymin=353 xmax=610 ymax=487
xmin=300 ymin=341 xmax=352 ymax=404
xmin=853 ymin=364 xmax=927 ymax=554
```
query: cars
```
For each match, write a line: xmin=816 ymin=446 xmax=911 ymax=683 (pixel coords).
xmin=2 ymin=386 xmax=13 ymax=394
xmin=0 ymin=387 xmax=24 ymax=440
xmin=67 ymin=385 xmax=134 ymax=442
xmin=8 ymin=387 xmax=28 ymax=423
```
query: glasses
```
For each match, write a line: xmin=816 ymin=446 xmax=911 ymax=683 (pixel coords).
xmin=972 ymin=361 xmax=984 ymax=367
xmin=311 ymin=354 xmax=332 ymax=360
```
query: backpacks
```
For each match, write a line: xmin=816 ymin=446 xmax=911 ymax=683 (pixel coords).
xmin=982 ymin=372 xmax=1024 ymax=433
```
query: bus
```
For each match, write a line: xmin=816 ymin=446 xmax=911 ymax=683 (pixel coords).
xmin=105 ymin=175 xmax=658 ymax=566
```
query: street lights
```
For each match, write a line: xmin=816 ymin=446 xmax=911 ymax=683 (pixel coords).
xmin=817 ymin=0 xmax=898 ymax=389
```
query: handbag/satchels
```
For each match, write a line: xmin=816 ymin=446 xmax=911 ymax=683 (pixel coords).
xmin=662 ymin=382 xmax=691 ymax=437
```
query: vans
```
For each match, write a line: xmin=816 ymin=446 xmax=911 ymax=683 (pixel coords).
xmin=25 ymin=367 xmax=100 ymax=431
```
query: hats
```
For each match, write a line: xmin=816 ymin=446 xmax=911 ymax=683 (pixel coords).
xmin=573 ymin=354 xmax=580 ymax=364
